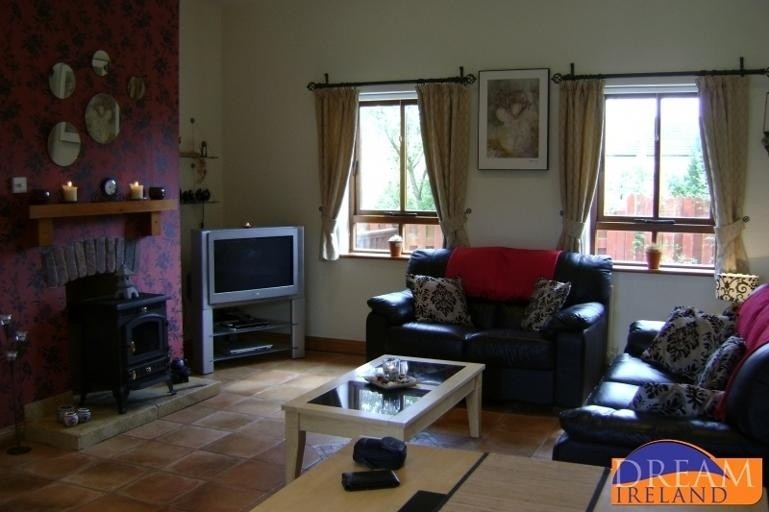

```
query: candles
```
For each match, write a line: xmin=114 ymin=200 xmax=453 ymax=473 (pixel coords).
xmin=60 ymin=181 xmax=76 ymax=204
xmin=127 ymin=178 xmax=143 ymax=201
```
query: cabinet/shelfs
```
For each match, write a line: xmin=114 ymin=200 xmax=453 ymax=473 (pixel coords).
xmin=190 ymin=295 xmax=307 ymax=374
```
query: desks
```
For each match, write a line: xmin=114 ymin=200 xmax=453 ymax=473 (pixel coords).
xmin=550 ymin=283 xmax=769 ymax=466
xmin=250 ymin=434 xmax=769 ymax=511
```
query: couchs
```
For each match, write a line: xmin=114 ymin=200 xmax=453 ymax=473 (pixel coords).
xmin=364 ymin=245 xmax=611 ymax=411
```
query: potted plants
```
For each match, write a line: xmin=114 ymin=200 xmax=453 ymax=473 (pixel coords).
xmin=388 ymin=234 xmax=402 ymax=260
xmin=646 ymin=243 xmax=661 ymax=271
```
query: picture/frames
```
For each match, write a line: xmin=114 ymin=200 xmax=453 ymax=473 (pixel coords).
xmin=90 ymin=49 xmax=113 ymax=79
xmin=84 ymin=93 xmax=121 ymax=143
xmin=130 ymin=75 xmax=144 ymax=100
xmin=46 ymin=122 xmax=82 ymax=166
xmin=478 ymin=67 xmax=549 ymax=170
xmin=46 ymin=61 xmax=77 ymax=99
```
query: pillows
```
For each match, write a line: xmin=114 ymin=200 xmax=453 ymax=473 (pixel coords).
xmin=630 ymin=376 xmax=725 ymax=423
xmin=641 ymin=305 xmax=739 ymax=382
xmin=407 ymin=273 xmax=469 ymax=326
xmin=695 ymin=336 xmax=745 ymax=397
xmin=519 ymin=273 xmax=570 ymax=334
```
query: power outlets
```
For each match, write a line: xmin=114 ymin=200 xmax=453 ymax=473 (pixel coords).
xmin=11 ymin=175 xmax=29 ymax=196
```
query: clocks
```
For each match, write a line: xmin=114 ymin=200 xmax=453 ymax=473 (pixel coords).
xmin=103 ymin=178 xmax=116 ymax=198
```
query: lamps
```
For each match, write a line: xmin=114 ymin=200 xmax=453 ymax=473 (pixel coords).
xmin=715 ymin=272 xmax=759 ymax=312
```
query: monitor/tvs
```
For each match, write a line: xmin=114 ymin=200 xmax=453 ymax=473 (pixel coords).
xmin=208 ymin=225 xmax=305 ymax=305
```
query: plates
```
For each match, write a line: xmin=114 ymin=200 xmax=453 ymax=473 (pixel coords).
xmin=368 ymin=375 xmax=417 ymax=389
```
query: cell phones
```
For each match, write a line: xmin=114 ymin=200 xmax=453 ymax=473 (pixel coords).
xmin=341 ymin=469 xmax=400 ymax=492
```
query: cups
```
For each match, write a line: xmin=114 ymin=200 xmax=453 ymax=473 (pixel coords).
xmin=383 ymin=358 xmax=402 ymax=381
xmin=388 ymin=234 xmax=404 ymax=258
xmin=644 ymin=243 xmax=663 ymax=270
